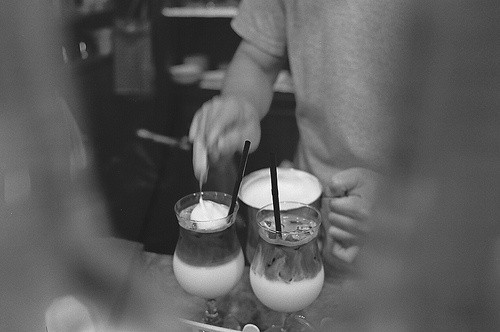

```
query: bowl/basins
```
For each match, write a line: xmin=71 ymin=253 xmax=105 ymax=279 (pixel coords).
xmin=170 ymin=54 xmax=227 ymax=89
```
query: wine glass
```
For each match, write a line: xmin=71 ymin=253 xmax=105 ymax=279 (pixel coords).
xmin=172 ymin=189 xmax=245 ymax=332
xmin=249 ymin=201 xmax=325 ymax=332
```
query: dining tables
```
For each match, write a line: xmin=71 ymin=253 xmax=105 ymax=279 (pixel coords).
xmin=109 ymin=238 xmax=352 ymax=332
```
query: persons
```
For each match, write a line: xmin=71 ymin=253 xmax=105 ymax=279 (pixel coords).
xmin=188 ymin=0 xmax=409 ymax=274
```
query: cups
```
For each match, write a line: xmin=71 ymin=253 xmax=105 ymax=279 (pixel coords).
xmin=237 ymin=165 xmax=334 ymax=265
